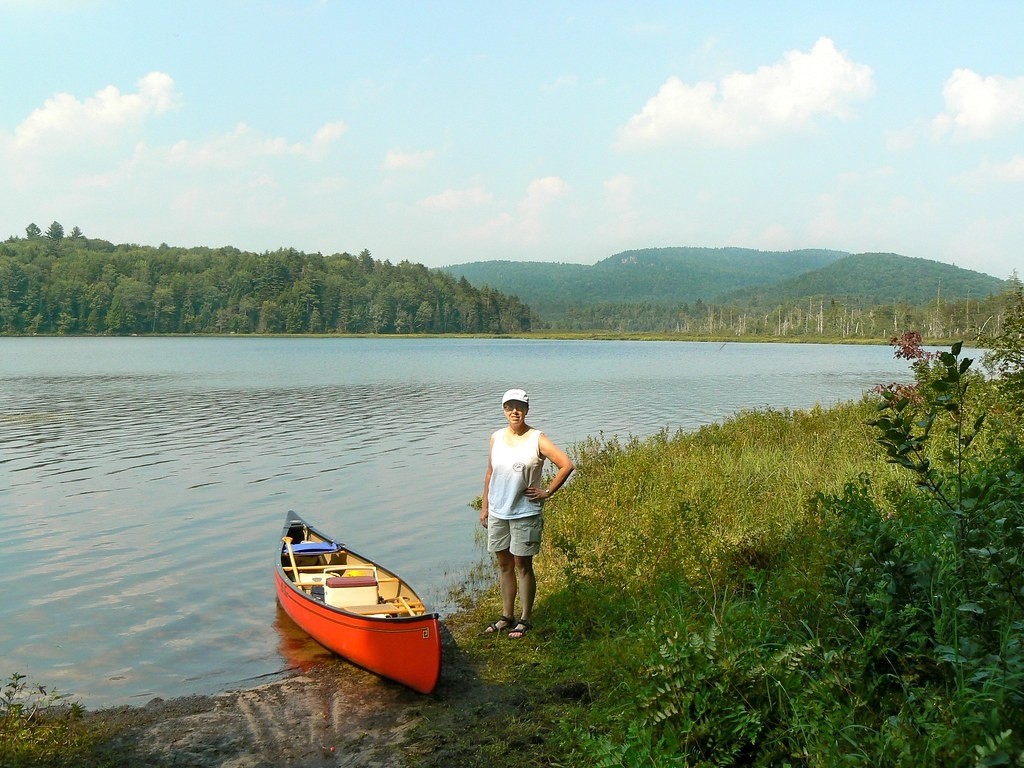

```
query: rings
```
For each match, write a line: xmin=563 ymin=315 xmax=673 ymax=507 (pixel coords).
xmin=534 ymin=493 xmax=536 ymax=496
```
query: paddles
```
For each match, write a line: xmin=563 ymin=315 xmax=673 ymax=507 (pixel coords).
xmin=282 ymin=536 xmax=303 ymax=590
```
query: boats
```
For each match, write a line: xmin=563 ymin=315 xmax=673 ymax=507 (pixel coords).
xmin=275 ymin=509 xmax=441 ymax=696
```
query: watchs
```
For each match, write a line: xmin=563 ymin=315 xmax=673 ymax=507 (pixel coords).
xmin=545 ymin=488 xmax=552 ymax=496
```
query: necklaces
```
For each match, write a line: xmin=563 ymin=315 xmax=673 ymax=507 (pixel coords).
xmin=510 ymin=424 xmax=525 ymax=435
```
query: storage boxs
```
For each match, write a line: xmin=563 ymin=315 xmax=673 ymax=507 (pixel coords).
xmin=311 ymin=587 xmax=325 ymax=602
xmin=323 ymin=567 xmax=381 ymax=609
xmin=299 ymin=573 xmax=335 ymax=595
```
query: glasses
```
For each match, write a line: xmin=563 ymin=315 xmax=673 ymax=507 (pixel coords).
xmin=504 ymin=402 xmax=528 ymax=412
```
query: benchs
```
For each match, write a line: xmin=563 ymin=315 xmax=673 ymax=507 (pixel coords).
xmin=282 ymin=542 xmax=346 ymax=557
xmin=345 ymin=603 xmax=423 ymax=614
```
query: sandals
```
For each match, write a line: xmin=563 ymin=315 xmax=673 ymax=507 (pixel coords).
xmin=507 ymin=620 xmax=533 ymax=640
xmin=484 ymin=616 xmax=515 ymax=635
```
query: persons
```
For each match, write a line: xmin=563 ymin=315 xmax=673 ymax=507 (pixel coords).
xmin=479 ymin=388 xmax=574 ymax=639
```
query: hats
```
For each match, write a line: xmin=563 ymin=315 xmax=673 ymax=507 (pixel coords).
xmin=502 ymin=389 xmax=529 ymax=409
